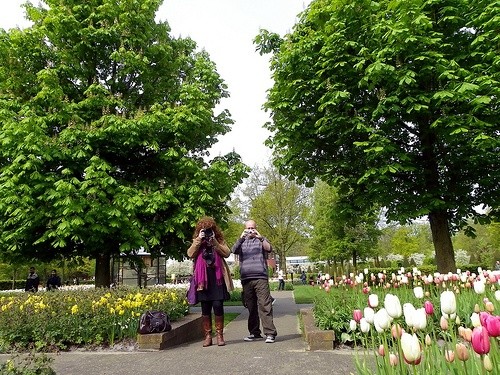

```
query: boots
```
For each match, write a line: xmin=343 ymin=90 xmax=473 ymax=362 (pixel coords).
xmin=202 ymin=315 xmax=213 ymax=347
xmin=215 ymin=316 xmax=225 ymax=346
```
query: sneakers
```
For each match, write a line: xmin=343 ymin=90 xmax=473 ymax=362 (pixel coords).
xmin=243 ymin=334 xmax=262 ymax=340
xmin=265 ymin=336 xmax=275 ymax=342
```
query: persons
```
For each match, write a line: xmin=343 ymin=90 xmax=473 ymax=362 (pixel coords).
xmin=187 ymin=217 xmax=234 ymax=347
xmin=278 ymin=268 xmax=285 ymax=290
xmin=25 ymin=267 xmax=40 ymax=293
xmin=47 ymin=269 xmax=61 ymax=291
xmin=232 ymin=220 xmax=278 ymax=343
xmin=301 ymin=271 xmax=306 ymax=285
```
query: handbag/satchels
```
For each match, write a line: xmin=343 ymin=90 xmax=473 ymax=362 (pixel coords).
xmin=140 ymin=310 xmax=172 ymax=334
xmin=185 ymin=278 xmax=200 ymax=305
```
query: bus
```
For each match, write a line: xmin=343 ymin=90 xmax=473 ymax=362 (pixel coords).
xmin=285 ymin=256 xmax=311 ymax=266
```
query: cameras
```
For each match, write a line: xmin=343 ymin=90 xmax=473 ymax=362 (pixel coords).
xmin=248 ymin=230 xmax=254 ymax=234
xmin=204 ymin=229 xmax=213 ymax=238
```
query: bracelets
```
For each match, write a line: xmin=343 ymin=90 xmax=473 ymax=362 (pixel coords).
xmin=260 ymin=237 xmax=264 ymax=242
